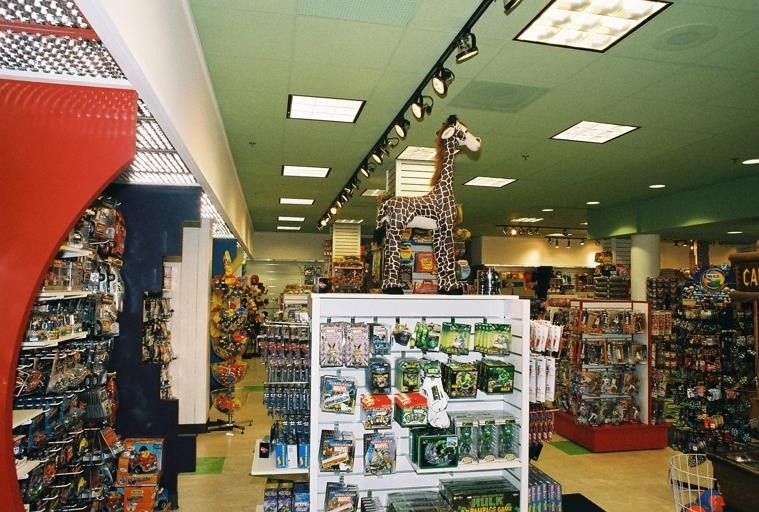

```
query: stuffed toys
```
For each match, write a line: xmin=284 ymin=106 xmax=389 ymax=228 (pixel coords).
xmin=373 ymin=112 xmax=483 ymax=295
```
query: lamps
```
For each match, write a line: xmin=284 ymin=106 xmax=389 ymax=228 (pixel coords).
xmin=500 ymin=226 xmax=585 ymax=248
xmin=359 ymin=0 xmax=524 ymax=180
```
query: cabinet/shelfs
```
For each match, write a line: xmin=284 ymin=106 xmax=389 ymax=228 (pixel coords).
xmin=550 ymin=277 xmax=596 ymax=288
xmin=10 ymin=191 xmax=127 ymax=512
xmin=309 ymin=292 xmax=531 ymax=512
xmin=547 ymin=296 xmax=673 ymax=453
